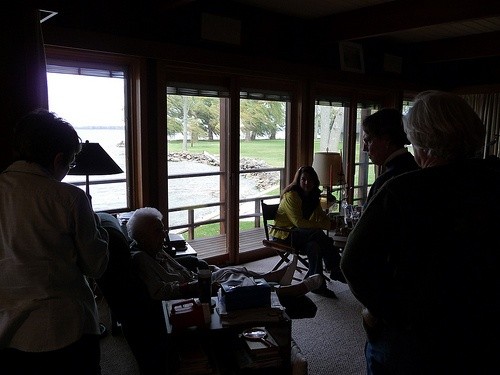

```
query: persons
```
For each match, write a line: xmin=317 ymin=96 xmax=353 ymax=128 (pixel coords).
xmin=340 ymin=90 xmax=500 ymax=375
xmin=269 ymin=165 xmax=347 ymax=290
xmin=0 ymin=108 xmax=110 ymax=375
xmin=125 ymin=207 xmax=324 ymax=303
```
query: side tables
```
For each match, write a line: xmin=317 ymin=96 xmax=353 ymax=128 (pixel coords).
xmin=162 ymin=295 xmax=291 ymax=375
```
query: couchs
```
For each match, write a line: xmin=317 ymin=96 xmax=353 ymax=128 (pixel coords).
xmin=91 ymin=212 xmax=209 ymax=374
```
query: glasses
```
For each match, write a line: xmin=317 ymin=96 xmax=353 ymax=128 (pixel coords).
xmin=70 ymin=162 xmax=77 ymax=169
xmin=362 ymin=134 xmax=377 ymax=146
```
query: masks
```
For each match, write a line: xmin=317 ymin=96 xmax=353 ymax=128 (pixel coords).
xmin=51 ymin=162 xmax=70 ymax=181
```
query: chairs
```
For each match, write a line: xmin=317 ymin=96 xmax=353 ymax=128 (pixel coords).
xmin=260 ymin=199 xmax=330 ymax=286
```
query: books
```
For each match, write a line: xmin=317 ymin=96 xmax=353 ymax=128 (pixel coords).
xmin=236 ymin=326 xmax=282 ymax=370
xmin=218 ymin=278 xmax=285 ymax=327
xmin=164 ymin=234 xmax=188 ymax=251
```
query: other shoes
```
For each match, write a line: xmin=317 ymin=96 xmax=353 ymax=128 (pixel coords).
xmin=279 ymin=254 xmax=298 ymax=286
xmin=330 ymin=272 xmax=348 ymax=283
xmin=289 ymin=274 xmax=323 ymax=294
xmin=311 ymin=289 xmax=336 ymax=299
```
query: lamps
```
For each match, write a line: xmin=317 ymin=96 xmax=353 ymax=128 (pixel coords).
xmin=68 ymin=139 xmax=124 ymax=201
xmin=311 ymin=152 xmax=347 ymax=237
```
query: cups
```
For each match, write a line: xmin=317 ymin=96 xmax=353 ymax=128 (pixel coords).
xmin=336 ymin=215 xmax=344 ymax=234
xmin=197 ymin=269 xmax=212 ymax=305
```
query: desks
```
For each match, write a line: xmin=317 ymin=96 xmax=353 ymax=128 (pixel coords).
xmin=334 ymin=233 xmax=349 ymax=246
